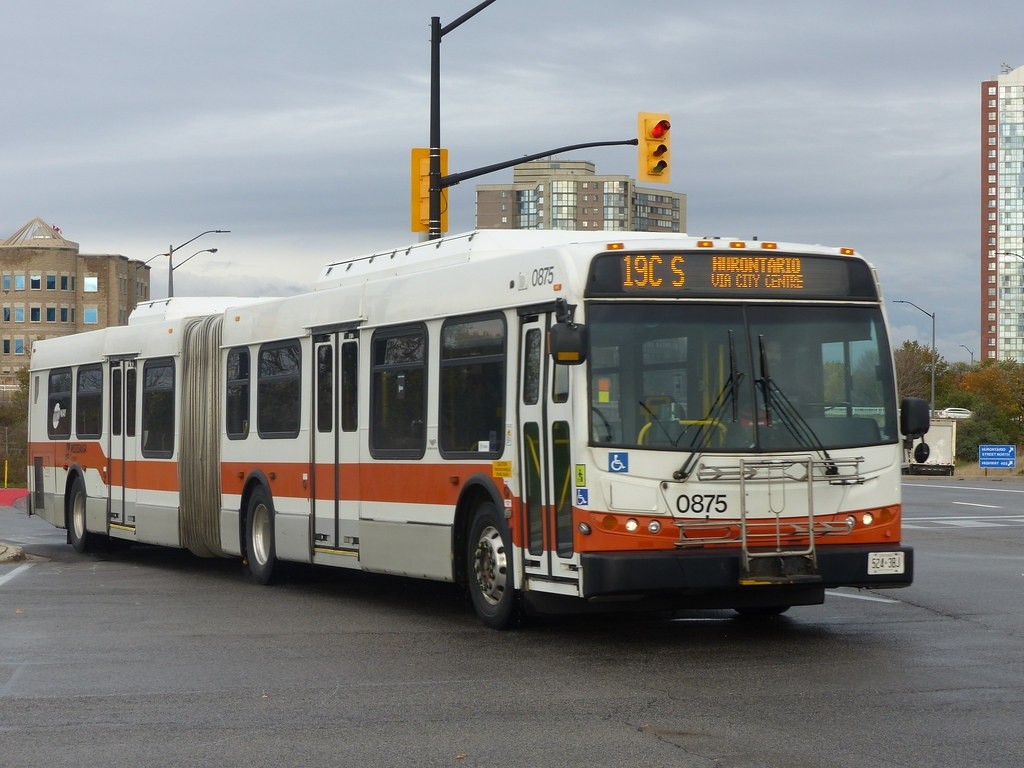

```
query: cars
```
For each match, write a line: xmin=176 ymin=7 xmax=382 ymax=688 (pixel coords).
xmin=938 ymin=407 xmax=973 ymax=419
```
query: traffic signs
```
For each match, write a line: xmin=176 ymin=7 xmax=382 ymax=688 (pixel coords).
xmin=978 ymin=444 xmax=1017 ymax=468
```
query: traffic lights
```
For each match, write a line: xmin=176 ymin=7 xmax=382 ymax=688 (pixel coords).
xmin=638 ymin=111 xmax=670 ymax=184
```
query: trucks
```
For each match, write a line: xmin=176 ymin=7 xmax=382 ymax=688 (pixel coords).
xmin=898 ymin=409 xmax=956 ymax=476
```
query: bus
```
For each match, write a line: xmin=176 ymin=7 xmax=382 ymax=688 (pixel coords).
xmin=21 ymin=228 xmax=932 ymax=630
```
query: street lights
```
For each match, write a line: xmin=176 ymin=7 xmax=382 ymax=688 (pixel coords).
xmin=168 ymin=230 xmax=231 ymax=298
xmin=892 ymin=300 xmax=936 ymax=419
